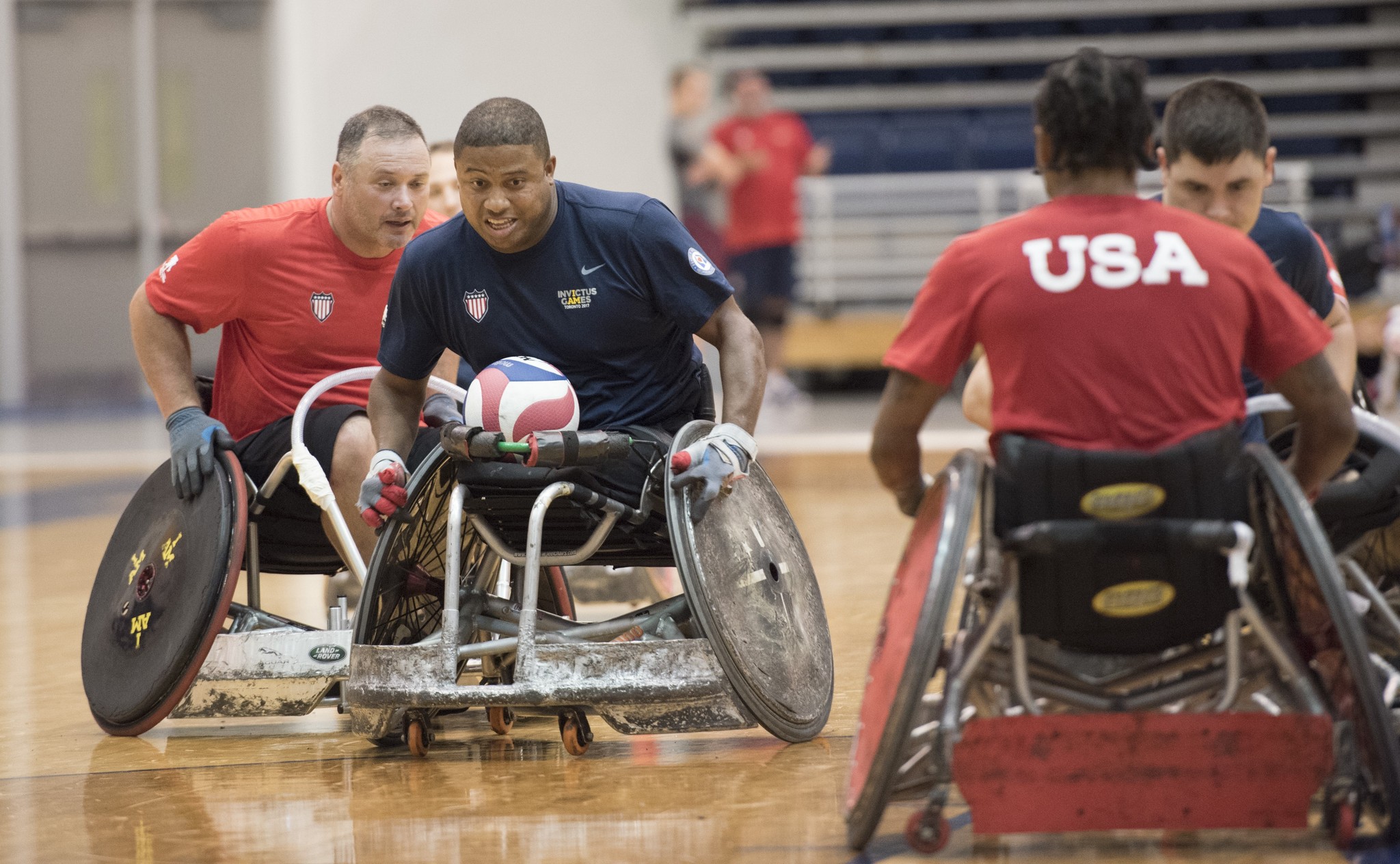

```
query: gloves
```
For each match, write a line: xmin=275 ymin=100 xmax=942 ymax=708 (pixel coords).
xmin=356 ymin=450 xmax=417 ymax=528
xmin=164 ymin=406 xmax=238 ymax=504
xmin=671 ymin=424 xmax=758 ymax=524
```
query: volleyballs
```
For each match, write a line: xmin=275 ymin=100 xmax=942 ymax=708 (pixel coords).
xmin=463 ymin=355 xmax=580 ymax=463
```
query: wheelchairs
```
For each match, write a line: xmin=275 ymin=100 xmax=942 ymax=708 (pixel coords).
xmin=840 ymin=385 xmax=1399 ymax=864
xmin=342 ymin=361 xmax=839 ymax=758
xmin=76 ymin=369 xmax=582 ymax=742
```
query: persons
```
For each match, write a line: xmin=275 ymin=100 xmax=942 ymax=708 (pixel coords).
xmin=961 ymin=78 xmax=1356 ymax=436
xmin=358 ymin=97 xmax=764 ymax=525
xmin=125 ymin=103 xmax=482 ymax=645
xmin=424 ymin=140 xmax=466 ymax=223
xmin=868 ymin=46 xmax=1359 ymax=517
xmin=671 ymin=65 xmax=837 ymax=371
